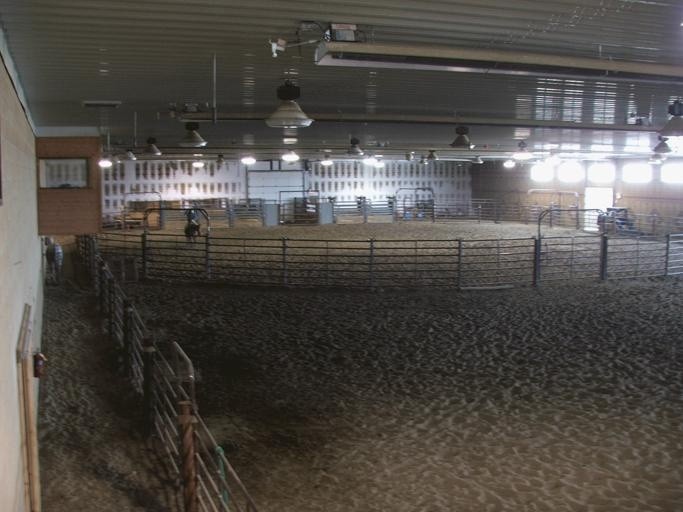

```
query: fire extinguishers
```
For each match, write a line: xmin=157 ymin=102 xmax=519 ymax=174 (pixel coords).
xmin=34 ymin=353 xmax=48 ymax=377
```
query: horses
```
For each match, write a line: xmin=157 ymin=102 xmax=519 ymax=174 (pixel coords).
xmin=182 ymin=223 xmax=202 ymax=243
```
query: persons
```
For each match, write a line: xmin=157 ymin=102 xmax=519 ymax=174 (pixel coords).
xmin=186 ymin=209 xmax=199 ymax=229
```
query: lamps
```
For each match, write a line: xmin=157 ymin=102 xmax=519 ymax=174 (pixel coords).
xmin=650 ymin=99 xmax=683 ymax=164
xmin=265 ymin=68 xmax=314 ymax=129
xmin=321 ymin=155 xmax=334 ymax=166
xmin=216 ymin=154 xmax=226 ymax=164
xmin=122 ymin=138 xmax=162 ymax=161
xmin=347 ymin=137 xmax=364 ymax=156
xmin=408 ymin=126 xmax=532 ymax=165
xmin=176 ymin=121 xmax=209 ymax=148
